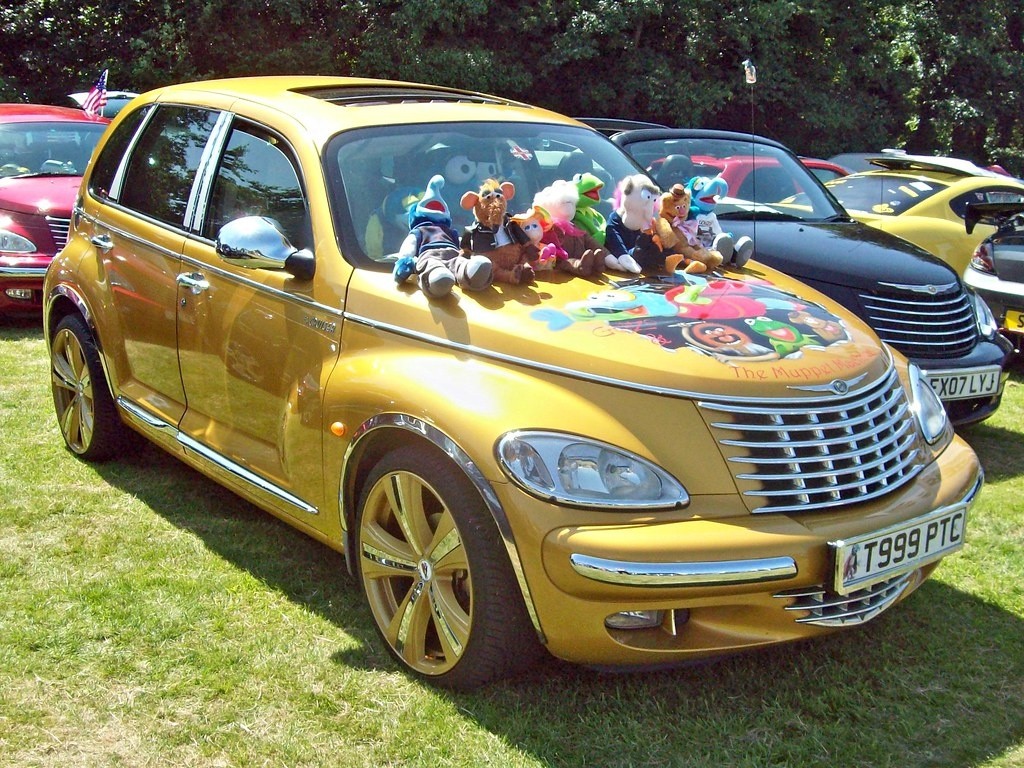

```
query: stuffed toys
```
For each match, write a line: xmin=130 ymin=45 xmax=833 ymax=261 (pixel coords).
xmin=364 ymin=186 xmax=423 ymax=259
xmin=685 ymin=176 xmax=753 ymax=270
xmin=603 ymin=174 xmax=660 ymax=274
xmin=570 ymin=170 xmax=607 ymax=246
xmin=392 ymin=174 xmax=494 ymax=299
xmin=531 ymin=180 xmax=626 ymax=278
xmin=458 ymin=179 xmax=541 ymax=286
xmin=424 ymin=148 xmax=496 ymax=225
xmin=641 ymin=184 xmax=723 ymax=275
xmin=510 ymin=205 xmax=569 ymax=271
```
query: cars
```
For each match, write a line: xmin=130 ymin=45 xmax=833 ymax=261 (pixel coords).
xmin=0 ymin=91 xmax=1024 ymax=428
xmin=43 ymin=73 xmax=985 ymax=689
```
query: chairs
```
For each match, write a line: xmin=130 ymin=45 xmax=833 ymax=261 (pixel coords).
xmin=28 ymin=141 xmax=84 ymax=168
xmin=417 ymin=146 xmax=479 ymax=236
xmin=303 ymin=144 xmax=392 ymax=259
xmin=657 ymin=154 xmax=696 ymax=192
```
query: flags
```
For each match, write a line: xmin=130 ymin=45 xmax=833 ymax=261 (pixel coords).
xmin=80 ymin=72 xmax=107 ymax=117
